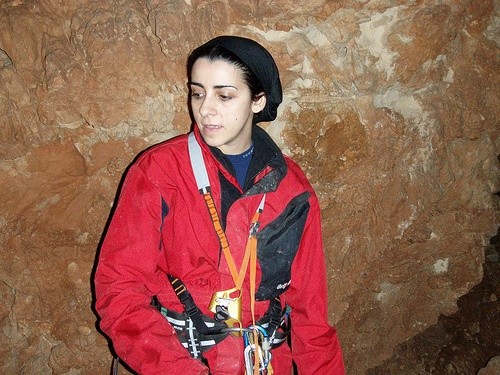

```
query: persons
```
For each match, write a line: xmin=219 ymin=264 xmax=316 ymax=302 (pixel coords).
xmin=92 ymin=35 xmax=347 ymax=375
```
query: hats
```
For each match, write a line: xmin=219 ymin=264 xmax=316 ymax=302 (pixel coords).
xmin=205 ymin=36 xmax=282 ymax=124
